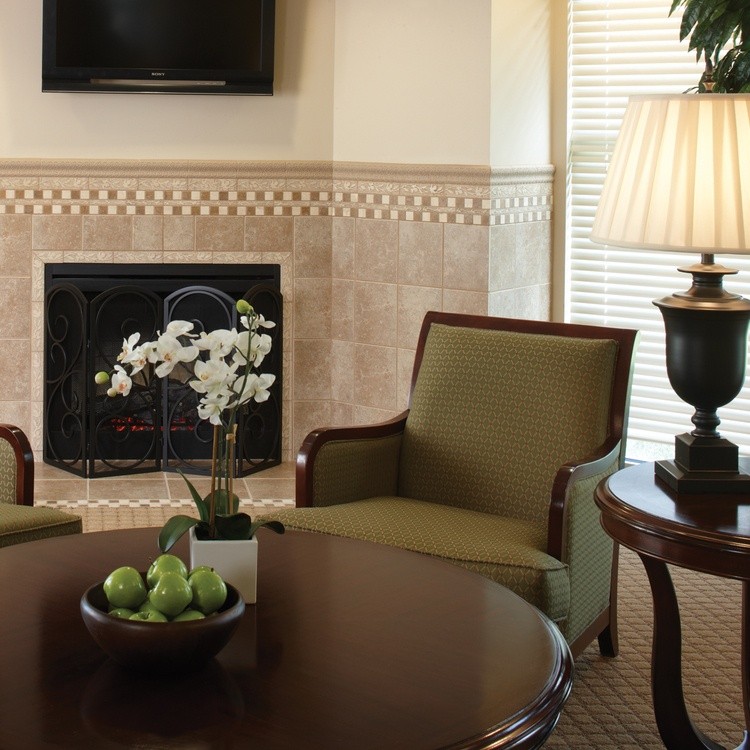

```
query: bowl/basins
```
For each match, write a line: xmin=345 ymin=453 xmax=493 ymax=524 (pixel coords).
xmin=80 ymin=572 xmax=247 ymax=674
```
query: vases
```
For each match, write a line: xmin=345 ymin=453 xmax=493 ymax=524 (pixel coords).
xmin=189 ymin=527 xmax=258 ymax=607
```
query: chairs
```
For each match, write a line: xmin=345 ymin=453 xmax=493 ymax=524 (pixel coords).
xmin=1 ymin=425 xmax=83 ymax=543
xmin=248 ymin=311 xmax=639 ymax=665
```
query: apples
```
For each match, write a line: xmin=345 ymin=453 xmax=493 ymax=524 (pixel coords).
xmin=102 ymin=553 xmax=228 ymax=622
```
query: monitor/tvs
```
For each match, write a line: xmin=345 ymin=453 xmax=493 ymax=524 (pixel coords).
xmin=42 ymin=0 xmax=276 ymax=97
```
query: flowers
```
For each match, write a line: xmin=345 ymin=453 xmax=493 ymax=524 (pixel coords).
xmin=94 ymin=298 xmax=286 ymax=552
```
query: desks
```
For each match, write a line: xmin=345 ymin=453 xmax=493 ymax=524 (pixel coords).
xmin=1 ymin=525 xmax=575 ymax=750
xmin=593 ymin=464 xmax=749 ymax=750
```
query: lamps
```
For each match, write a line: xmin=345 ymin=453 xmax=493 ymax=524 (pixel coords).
xmin=589 ymin=94 xmax=750 ymax=499
